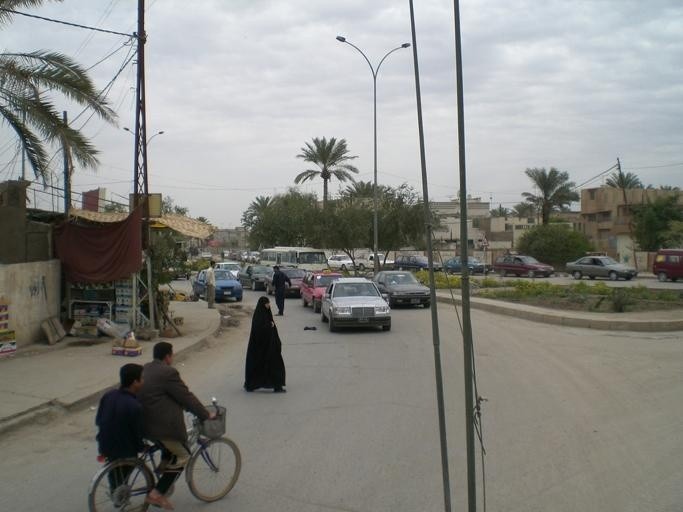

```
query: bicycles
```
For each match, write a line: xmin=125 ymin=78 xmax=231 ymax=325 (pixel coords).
xmin=87 ymin=395 xmax=242 ymax=512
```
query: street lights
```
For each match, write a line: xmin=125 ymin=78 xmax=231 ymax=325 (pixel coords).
xmin=336 ymin=35 xmax=411 ymax=277
xmin=123 ymin=127 xmax=164 ymax=147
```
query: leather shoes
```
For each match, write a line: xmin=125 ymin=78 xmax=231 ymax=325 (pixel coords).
xmin=145 ymin=491 xmax=174 ymax=512
xmin=208 ymin=305 xmax=216 ymax=309
xmin=246 ymin=387 xmax=260 ymax=392
xmin=274 ymin=388 xmax=288 ymax=394
xmin=275 ymin=312 xmax=284 ymax=317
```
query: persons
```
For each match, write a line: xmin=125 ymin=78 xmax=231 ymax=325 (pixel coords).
xmin=95 ymin=363 xmax=148 ymax=510
xmin=205 ymin=260 xmax=216 ymax=309
xmin=271 ymin=266 xmax=292 ymax=317
xmin=243 ymin=296 xmax=287 ymax=392
xmin=135 ymin=342 xmax=217 ymax=511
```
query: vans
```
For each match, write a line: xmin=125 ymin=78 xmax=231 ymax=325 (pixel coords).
xmin=653 ymin=249 xmax=683 ymax=282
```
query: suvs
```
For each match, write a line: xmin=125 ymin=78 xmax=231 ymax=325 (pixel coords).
xmin=327 ymin=255 xmax=356 ymax=271
xmin=392 ymin=255 xmax=443 ymax=273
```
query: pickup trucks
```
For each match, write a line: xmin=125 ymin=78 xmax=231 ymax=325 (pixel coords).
xmin=354 ymin=254 xmax=387 ymax=271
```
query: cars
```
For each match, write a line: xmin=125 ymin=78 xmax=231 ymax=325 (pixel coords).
xmin=443 ymin=256 xmax=489 ymax=275
xmin=264 ymin=269 xmax=306 ymax=298
xmin=494 ymin=255 xmax=554 ymax=278
xmin=321 ymin=277 xmax=391 ymax=331
xmin=237 ymin=265 xmax=274 ymax=290
xmin=191 ymin=269 xmax=243 ymax=302
xmin=215 ymin=263 xmax=242 ymax=278
xmin=300 ymin=270 xmax=342 ymax=313
xmin=566 ymin=256 xmax=638 ymax=281
xmin=373 ymin=270 xmax=432 ymax=309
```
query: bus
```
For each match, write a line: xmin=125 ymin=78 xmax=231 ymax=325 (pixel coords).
xmin=259 ymin=246 xmax=330 ymax=272
